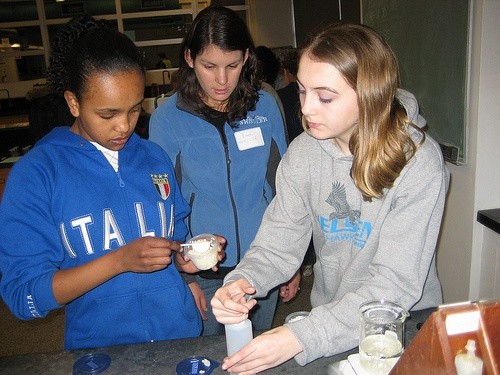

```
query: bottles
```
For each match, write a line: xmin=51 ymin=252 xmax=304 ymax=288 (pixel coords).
xmin=454 ymin=339 xmax=483 ymax=375
xmin=225 ymin=319 xmax=257 ymax=375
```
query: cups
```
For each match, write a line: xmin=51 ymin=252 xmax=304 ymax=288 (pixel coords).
xmin=358 ymin=298 xmax=410 ymax=374
xmin=184 ymin=233 xmax=218 ymax=270
xmin=285 ymin=311 xmax=310 ymax=324
